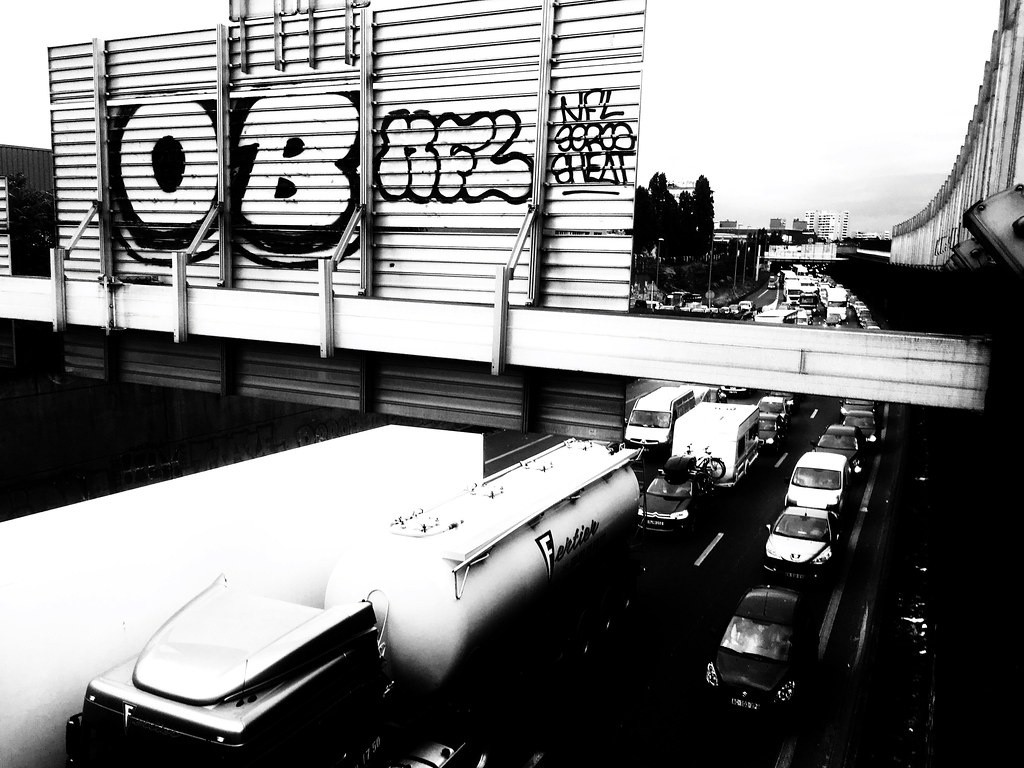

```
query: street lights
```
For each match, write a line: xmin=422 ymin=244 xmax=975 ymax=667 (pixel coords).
xmin=733 ymin=224 xmax=743 ymax=292
xmin=708 ymin=222 xmax=719 ymax=307
xmin=656 ymin=238 xmax=663 ymax=285
xmin=747 ymin=226 xmax=751 ymax=285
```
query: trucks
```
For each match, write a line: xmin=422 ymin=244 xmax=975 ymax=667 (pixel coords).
xmin=792 ymin=264 xmax=808 ymax=277
xmin=624 ymin=385 xmax=712 ymax=457
xmin=784 ymin=280 xmax=803 ymax=304
xmin=779 ymin=270 xmax=797 ymax=287
xmin=798 ymin=276 xmax=813 ymax=286
xmin=800 ymin=293 xmax=818 ymax=315
xmin=672 ymin=401 xmax=764 ymax=488
xmin=825 ymin=288 xmax=850 ymax=322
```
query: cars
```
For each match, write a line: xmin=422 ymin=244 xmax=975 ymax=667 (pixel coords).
xmin=762 ymin=507 xmax=840 ymax=586
xmin=637 ymin=457 xmax=713 ymax=540
xmin=842 ymin=411 xmax=878 ymax=445
xmin=702 ymin=584 xmax=818 ymax=729
xmin=768 ymin=263 xmax=880 ymax=330
xmin=646 ymin=301 xmax=753 ymax=315
xmin=757 ymin=412 xmax=786 ymax=454
xmin=839 ymin=399 xmax=877 ymax=415
xmin=811 ymin=424 xmax=864 ymax=463
xmin=784 ymin=450 xmax=852 ymax=516
xmin=759 ymin=396 xmax=791 ymax=426
xmin=768 ymin=391 xmax=793 ymax=407
xmin=720 ymin=385 xmax=747 ymax=393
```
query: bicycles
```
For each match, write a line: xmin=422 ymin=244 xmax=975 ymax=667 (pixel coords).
xmin=684 ymin=443 xmax=726 ymax=480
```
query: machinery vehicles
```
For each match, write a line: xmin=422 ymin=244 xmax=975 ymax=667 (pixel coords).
xmin=64 ymin=439 xmax=646 ymax=768
